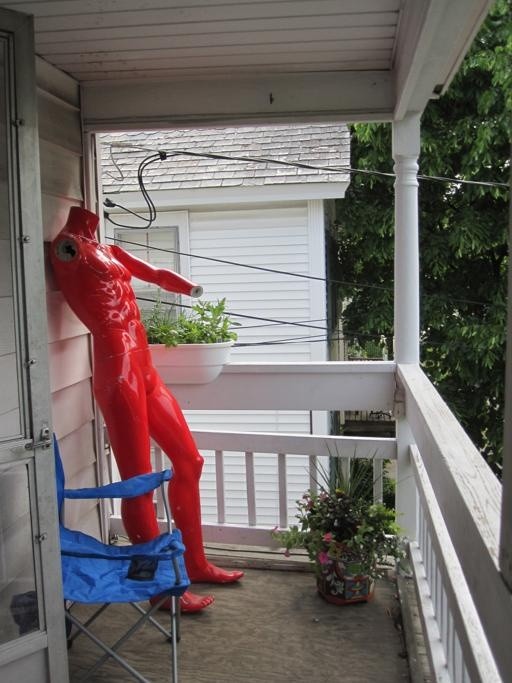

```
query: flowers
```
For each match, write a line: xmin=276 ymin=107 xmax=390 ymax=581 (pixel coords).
xmin=265 ymin=435 xmax=414 ymax=584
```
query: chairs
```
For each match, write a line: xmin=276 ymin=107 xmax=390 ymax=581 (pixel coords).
xmin=44 ymin=430 xmax=194 ymax=683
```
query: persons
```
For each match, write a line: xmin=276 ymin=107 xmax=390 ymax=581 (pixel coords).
xmin=49 ymin=203 xmax=247 ymax=612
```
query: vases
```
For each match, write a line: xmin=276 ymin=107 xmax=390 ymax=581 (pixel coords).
xmin=311 ymin=547 xmax=377 ymax=606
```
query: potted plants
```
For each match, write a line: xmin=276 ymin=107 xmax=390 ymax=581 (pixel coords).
xmin=127 ymin=283 xmax=238 ymax=385
